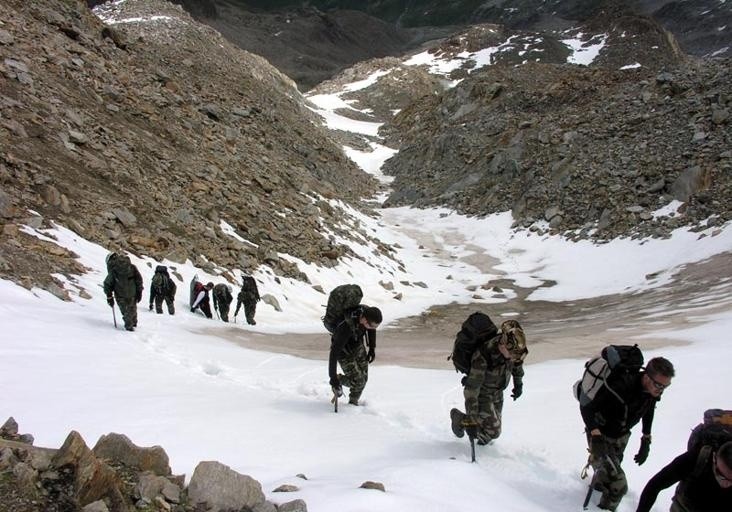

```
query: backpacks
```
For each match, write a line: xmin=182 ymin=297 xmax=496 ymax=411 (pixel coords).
xmin=678 ymin=409 xmax=732 ymax=491
xmin=194 ymin=282 xmax=208 ymax=304
xmin=572 ymin=345 xmax=643 ymax=405
xmin=106 ymin=252 xmax=118 ymax=271
xmin=156 ymin=266 xmax=169 ymax=278
xmin=451 ymin=312 xmax=497 ymax=374
xmin=242 ymin=279 xmax=259 ymax=300
xmin=325 ymin=284 xmax=363 ymax=333
xmin=216 ymin=283 xmax=233 ymax=303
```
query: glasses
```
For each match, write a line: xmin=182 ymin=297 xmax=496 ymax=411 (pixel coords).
xmin=714 ymin=457 xmax=732 ymax=482
xmin=649 ymin=376 xmax=671 ymax=389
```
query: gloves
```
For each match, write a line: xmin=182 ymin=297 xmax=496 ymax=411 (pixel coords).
xmin=367 ymin=349 xmax=375 ymax=363
xmin=330 ymin=378 xmax=339 ymax=385
xmin=511 ymin=385 xmax=521 ymax=401
xmin=590 ymin=436 xmax=604 ymax=459
xmin=465 ymin=398 xmax=478 ymax=415
xmin=108 ymin=298 xmax=114 ymax=306
xmin=634 ymin=438 xmax=650 ymax=465
xmin=136 ymin=291 xmax=142 ymax=302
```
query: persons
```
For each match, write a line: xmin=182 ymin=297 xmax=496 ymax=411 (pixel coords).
xmin=148 ymin=274 xmax=177 ymax=315
xmin=637 ymin=439 xmax=730 ymax=511
xmin=191 ymin=281 xmax=215 ymax=318
xmin=234 ymin=291 xmax=257 ymax=326
xmin=213 ymin=287 xmax=232 ymax=322
xmin=448 ymin=319 xmax=529 ymax=445
xmin=329 ymin=305 xmax=382 ymax=405
xmin=584 ymin=357 xmax=674 ymax=511
xmin=103 ymin=257 xmax=143 ymax=332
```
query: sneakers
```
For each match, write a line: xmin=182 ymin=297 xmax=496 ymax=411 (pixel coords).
xmin=332 ymin=386 xmax=342 ymax=396
xmin=450 ymin=409 xmax=465 ymax=437
